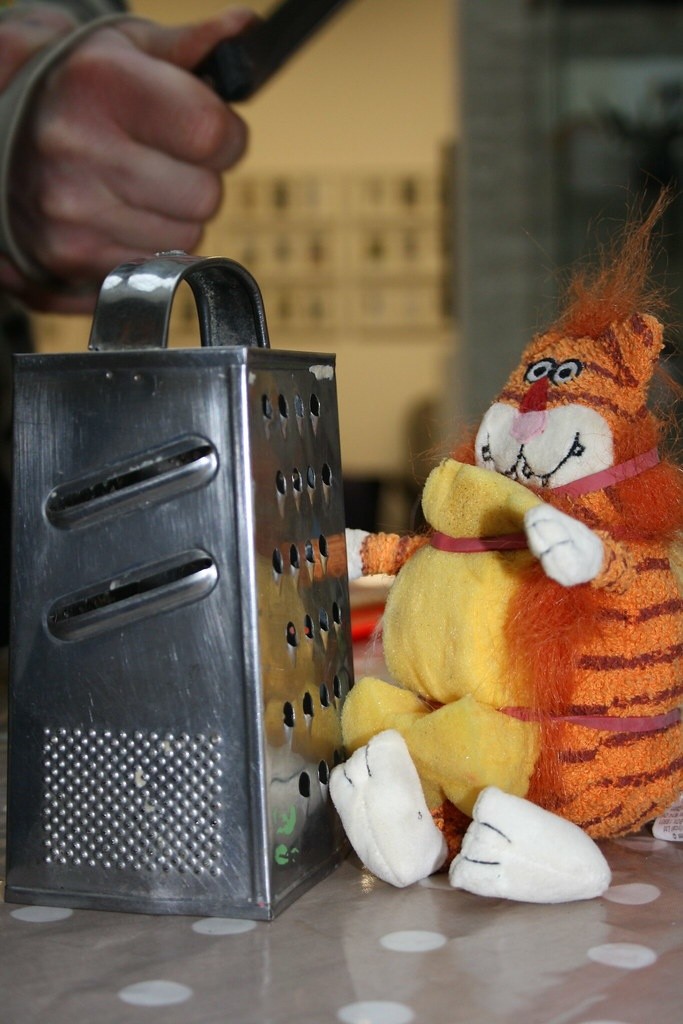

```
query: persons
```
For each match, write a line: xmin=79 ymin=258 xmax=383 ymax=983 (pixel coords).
xmin=2 ymin=0 xmax=260 ymax=312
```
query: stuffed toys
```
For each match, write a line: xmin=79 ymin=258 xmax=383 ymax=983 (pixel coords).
xmin=316 ymin=186 xmax=683 ymax=903
